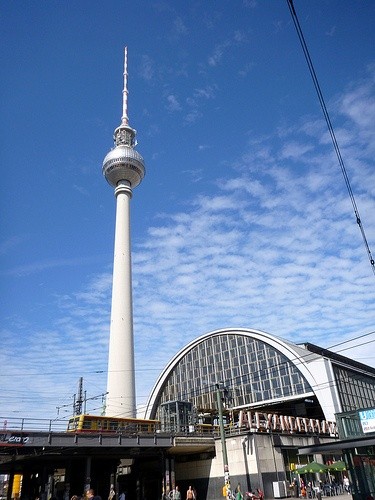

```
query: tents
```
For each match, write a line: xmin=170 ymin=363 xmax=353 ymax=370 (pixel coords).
xmin=294 ymin=460 xmax=350 ymax=488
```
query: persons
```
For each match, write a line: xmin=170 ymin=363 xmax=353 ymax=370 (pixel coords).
xmin=235 ymin=482 xmax=262 ymax=500
xmin=71 ymin=489 xmax=96 ymax=500
xmin=187 ymin=486 xmax=195 ymax=500
xmin=300 ymin=480 xmax=316 ymax=499
xmin=344 ymin=476 xmax=351 ymax=495
xmin=175 ymin=486 xmax=180 ymax=500
